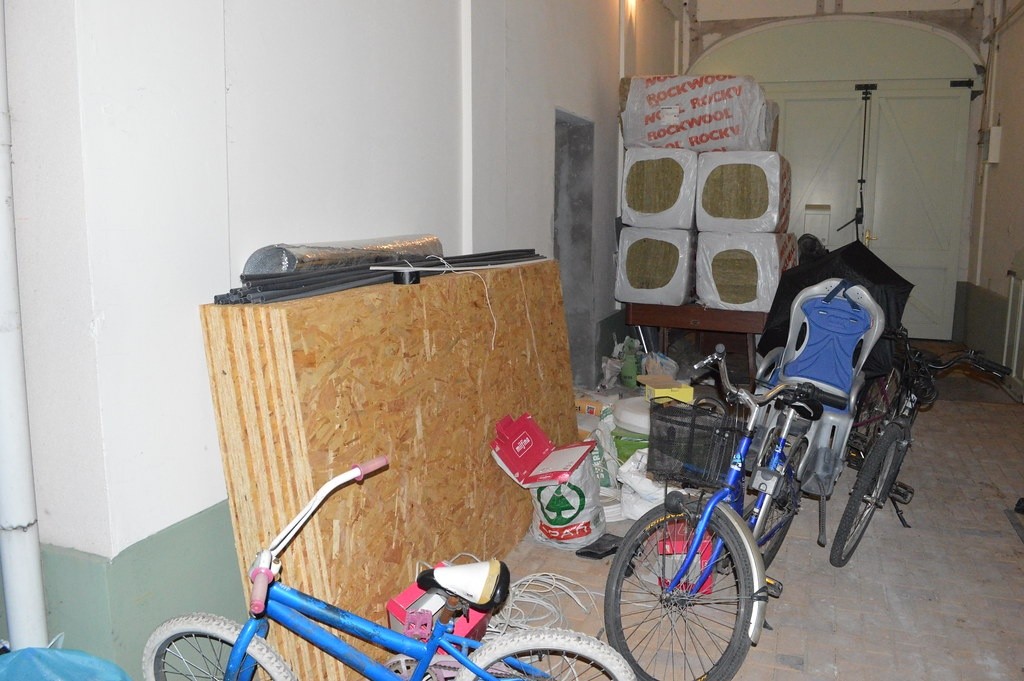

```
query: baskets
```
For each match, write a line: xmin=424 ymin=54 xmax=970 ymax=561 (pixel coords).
xmin=648 ymin=396 xmax=757 ymax=488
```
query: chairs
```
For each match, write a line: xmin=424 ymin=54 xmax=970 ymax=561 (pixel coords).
xmin=745 ymin=276 xmax=884 ymax=495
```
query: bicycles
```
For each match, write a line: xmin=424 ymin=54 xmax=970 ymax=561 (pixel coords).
xmin=142 ymin=459 xmax=646 ymax=681
xmin=600 ymin=342 xmax=826 ymax=681
xmin=830 ymin=321 xmax=1014 ymax=570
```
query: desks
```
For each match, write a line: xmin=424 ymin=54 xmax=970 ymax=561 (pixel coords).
xmin=627 ymin=302 xmax=770 ymax=395
